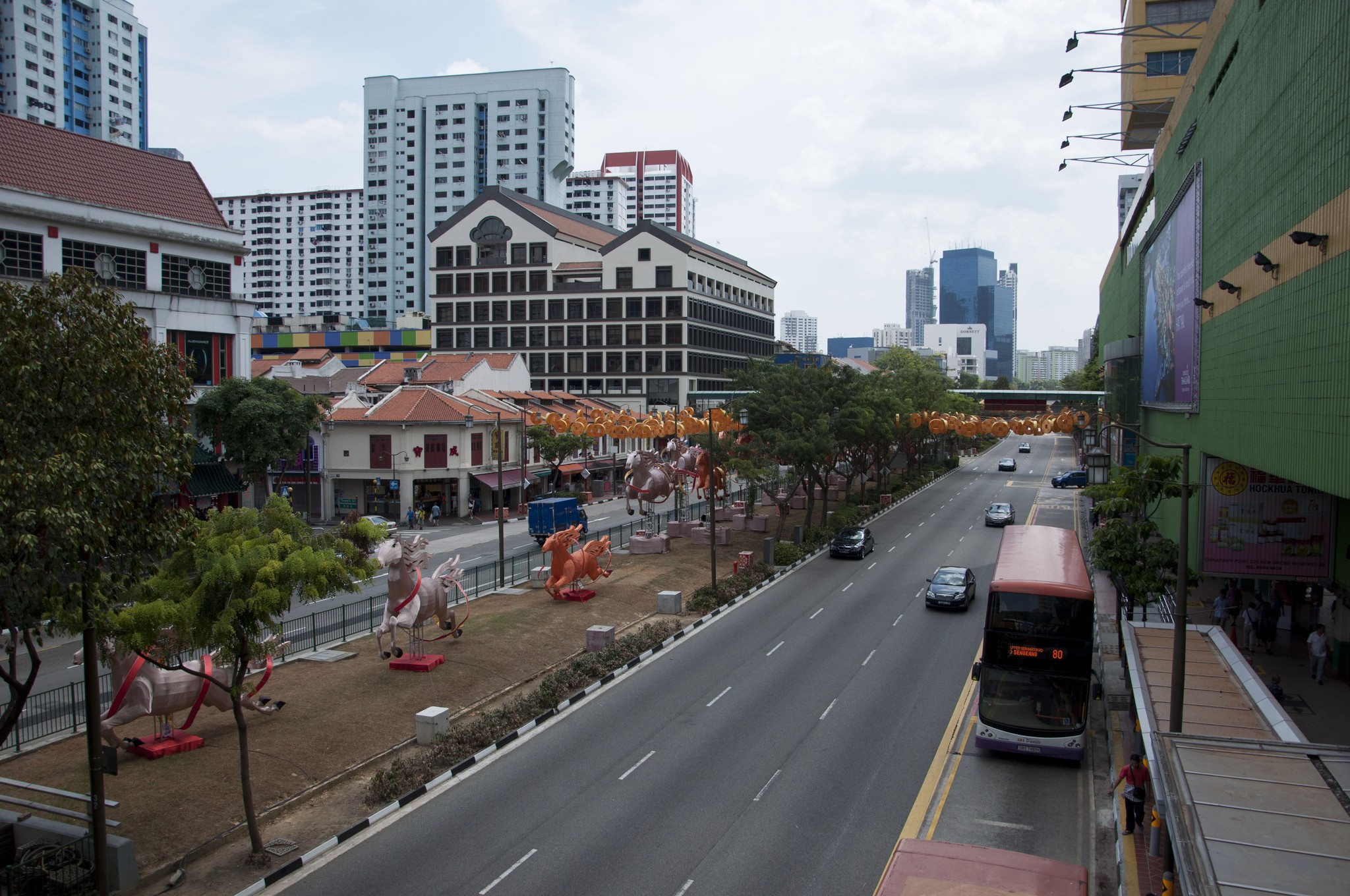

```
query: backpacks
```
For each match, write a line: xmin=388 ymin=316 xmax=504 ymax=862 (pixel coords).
xmin=1271 ymin=686 xmax=1283 ymax=703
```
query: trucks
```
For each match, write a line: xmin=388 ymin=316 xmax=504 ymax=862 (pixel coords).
xmin=528 ymin=497 xmax=588 ymax=546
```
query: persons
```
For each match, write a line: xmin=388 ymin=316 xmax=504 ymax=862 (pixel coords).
xmin=1107 ymin=753 xmax=1151 ymax=835
xmin=1081 ymin=464 xmax=1085 ymax=471
xmin=1092 ymin=505 xmax=1098 ymax=531
xmin=202 ymin=508 xmax=211 ymax=521
xmin=1246 ymin=656 xmax=1255 ymax=670
xmin=405 ymin=507 xmax=425 ymax=530
xmin=468 ymin=489 xmax=481 ymax=519
xmin=1224 ymin=578 xmax=1284 ymax=655
xmin=340 ymin=517 xmax=346 ymax=529
xmin=452 ymin=493 xmax=458 ymax=514
xmin=549 ymin=481 xmax=553 ymax=492
xmin=564 ymin=480 xmax=570 ymax=492
xmin=425 ymin=490 xmax=431 ymax=499
xmin=526 ymin=485 xmax=532 ymax=502
xmin=1208 ymin=588 xmax=1231 ymax=631
xmin=1307 ymin=624 xmax=1330 ymax=686
xmin=439 ymin=493 xmax=449 ymax=516
xmin=429 ymin=502 xmax=441 ymax=526
xmin=503 ymin=489 xmax=509 ymax=504
xmin=604 ymin=473 xmax=609 ymax=481
xmin=1266 ymin=674 xmax=1285 ymax=708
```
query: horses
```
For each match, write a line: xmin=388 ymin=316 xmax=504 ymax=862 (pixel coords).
xmin=664 ymin=436 xmax=730 ymax=501
xmin=375 ymin=533 xmax=469 ymax=660
xmin=542 ymin=524 xmax=613 ymax=600
xmin=624 ymin=447 xmax=685 ymax=515
xmin=72 ymin=624 xmax=291 ymax=749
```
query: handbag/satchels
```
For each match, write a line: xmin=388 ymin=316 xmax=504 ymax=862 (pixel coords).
xmin=1133 ymin=787 xmax=1147 ymax=800
xmin=429 ymin=514 xmax=433 ymax=522
xmin=1252 ymin=621 xmax=1259 ymax=630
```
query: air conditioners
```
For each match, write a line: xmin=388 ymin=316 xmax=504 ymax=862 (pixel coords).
xmin=213 ymin=99 xmax=670 ymax=308
xmin=0 ymin=8 xmax=95 ymax=120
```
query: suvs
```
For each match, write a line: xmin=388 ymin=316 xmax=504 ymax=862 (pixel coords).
xmin=1051 ymin=470 xmax=1086 ymax=489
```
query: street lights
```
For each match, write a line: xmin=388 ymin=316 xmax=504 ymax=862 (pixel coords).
xmin=708 ymin=399 xmax=749 ymax=593
xmin=613 ymin=404 xmax=631 ymax=495
xmin=521 ymin=405 xmax=542 ymax=516
xmin=1085 ymin=424 xmax=1192 ymax=733
xmin=1077 ymin=406 xmax=1142 ymax=620
xmin=651 ymin=400 xmax=678 ymax=521
xmin=306 ymin=411 xmax=335 ymax=526
xmin=379 ymin=451 xmax=410 ymax=523
xmin=464 ymin=405 xmax=504 ymax=588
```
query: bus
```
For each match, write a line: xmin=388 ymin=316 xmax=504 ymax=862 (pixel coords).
xmin=971 ymin=525 xmax=1103 ymax=761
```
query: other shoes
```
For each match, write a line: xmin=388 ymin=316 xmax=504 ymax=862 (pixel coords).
xmin=1230 ymin=621 xmax=1236 ymax=626
xmin=1137 ymin=822 xmax=1145 ymax=831
xmin=1267 ymin=648 xmax=1273 ymax=656
xmin=1121 ymin=829 xmax=1133 ymax=835
xmin=1310 ymin=674 xmax=1316 ymax=680
xmin=470 ymin=517 xmax=471 ymax=518
xmin=1317 ymin=680 xmax=1323 ymax=685
xmin=409 ymin=528 xmax=411 ymax=530
xmin=1250 ymin=649 xmax=1255 ymax=652
xmin=471 ymin=516 xmax=473 ymax=519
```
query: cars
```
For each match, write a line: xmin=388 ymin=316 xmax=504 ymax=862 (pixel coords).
xmin=360 ymin=515 xmax=398 ymax=537
xmin=985 ymin=502 xmax=1015 ymax=527
xmin=829 ymin=526 xmax=874 ymax=560
xmin=998 ymin=458 xmax=1017 ymax=472
xmin=1019 ymin=443 xmax=1031 ymax=453
xmin=925 ymin=566 xmax=977 ymax=612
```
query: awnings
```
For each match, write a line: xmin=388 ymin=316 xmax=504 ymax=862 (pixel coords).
xmin=474 ymin=468 xmax=541 ymax=490
xmin=558 ymin=458 xmax=627 ymax=475
xmin=526 ymin=467 xmax=552 ymax=476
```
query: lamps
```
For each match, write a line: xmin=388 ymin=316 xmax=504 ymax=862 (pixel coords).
xmin=1218 ymin=280 xmax=1241 ymax=299
xmin=1287 ymin=231 xmax=1328 ymax=253
xmin=1058 ymin=154 xmax=1149 ymax=171
xmin=1061 ymin=128 xmax=1161 ymax=149
xmin=1255 ymin=252 xmax=1280 ymax=279
xmin=1066 ymin=15 xmax=1210 ymax=53
xmin=1192 ymin=298 xmax=1214 ymax=316
xmin=1059 ymin=55 xmax=1195 ymax=88
xmin=1127 ymin=334 xmax=1136 ymax=338
xmin=1062 ymin=96 xmax=1174 ymax=121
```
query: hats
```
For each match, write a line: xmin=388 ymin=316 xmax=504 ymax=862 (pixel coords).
xmin=418 ymin=506 xmax=422 ymax=509
